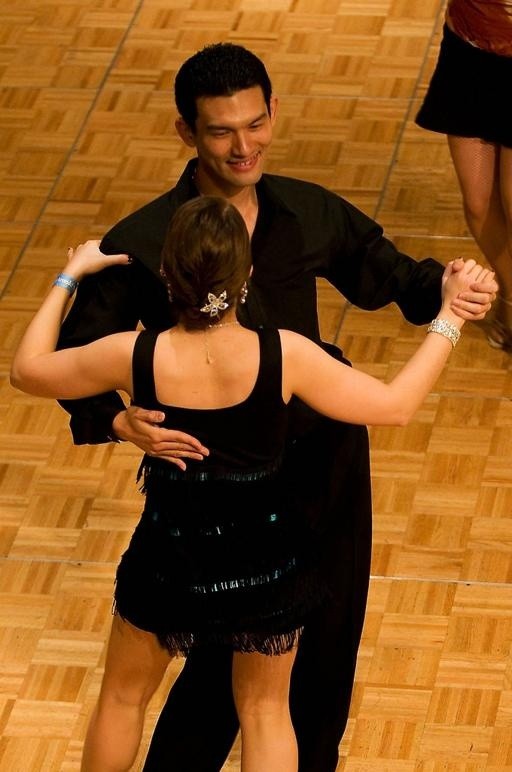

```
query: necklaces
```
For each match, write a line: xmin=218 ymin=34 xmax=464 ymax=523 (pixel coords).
xmin=202 ymin=322 xmax=242 ymax=329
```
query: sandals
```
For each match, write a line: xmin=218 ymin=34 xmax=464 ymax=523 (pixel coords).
xmin=488 ymin=294 xmax=511 ymax=349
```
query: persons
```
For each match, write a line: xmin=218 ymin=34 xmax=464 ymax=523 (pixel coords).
xmin=409 ymin=1 xmax=512 ymax=352
xmin=8 ymin=192 xmax=504 ymax=772
xmin=54 ymin=40 xmax=500 ymax=771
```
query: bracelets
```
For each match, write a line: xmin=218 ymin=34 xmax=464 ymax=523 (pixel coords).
xmin=425 ymin=319 xmax=463 ymax=349
xmin=53 ymin=273 xmax=81 ymax=297
